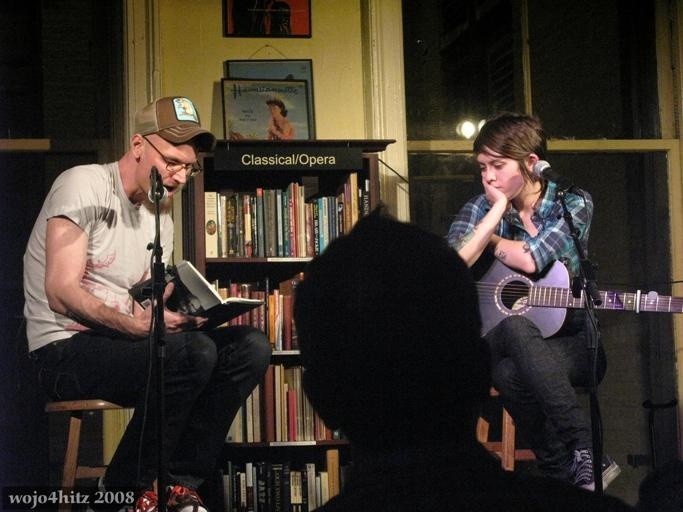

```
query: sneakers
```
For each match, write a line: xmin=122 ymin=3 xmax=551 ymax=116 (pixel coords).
xmin=118 ymin=484 xmax=210 ymax=512
xmin=570 ymin=447 xmax=622 ymax=492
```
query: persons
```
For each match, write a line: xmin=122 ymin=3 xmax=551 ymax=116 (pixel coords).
xmin=445 ymin=110 xmax=621 ymax=493
xmin=19 ymin=93 xmax=273 ymax=511
xmin=263 ymin=98 xmax=293 ymax=141
xmin=290 ymin=201 xmax=643 ymax=511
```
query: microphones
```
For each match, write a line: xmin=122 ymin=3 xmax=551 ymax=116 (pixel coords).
xmin=149 ymin=163 xmax=169 ymax=203
xmin=533 ymin=160 xmax=587 ymax=202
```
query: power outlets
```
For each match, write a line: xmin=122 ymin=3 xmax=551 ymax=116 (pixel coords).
xmin=181 ymin=138 xmax=397 ymax=512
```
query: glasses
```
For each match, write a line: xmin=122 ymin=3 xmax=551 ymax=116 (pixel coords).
xmin=143 ymin=137 xmax=203 ymax=178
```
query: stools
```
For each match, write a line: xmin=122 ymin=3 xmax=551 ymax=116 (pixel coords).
xmin=475 ymin=386 xmax=587 ymax=470
xmin=46 ymin=400 xmax=122 ymax=512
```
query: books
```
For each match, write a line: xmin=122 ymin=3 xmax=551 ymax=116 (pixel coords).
xmin=125 ymin=261 xmax=265 ymax=333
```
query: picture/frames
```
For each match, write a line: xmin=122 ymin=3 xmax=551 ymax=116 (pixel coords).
xmin=225 ymin=0 xmax=312 ymax=38
xmin=227 ymin=59 xmax=316 ymax=139
xmin=221 ymin=77 xmax=310 ymax=140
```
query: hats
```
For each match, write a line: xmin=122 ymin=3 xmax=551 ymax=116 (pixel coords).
xmin=133 ymin=95 xmax=218 ymax=154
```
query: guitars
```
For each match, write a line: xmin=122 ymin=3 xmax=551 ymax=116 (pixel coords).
xmin=475 ymin=259 xmax=683 ymax=338
